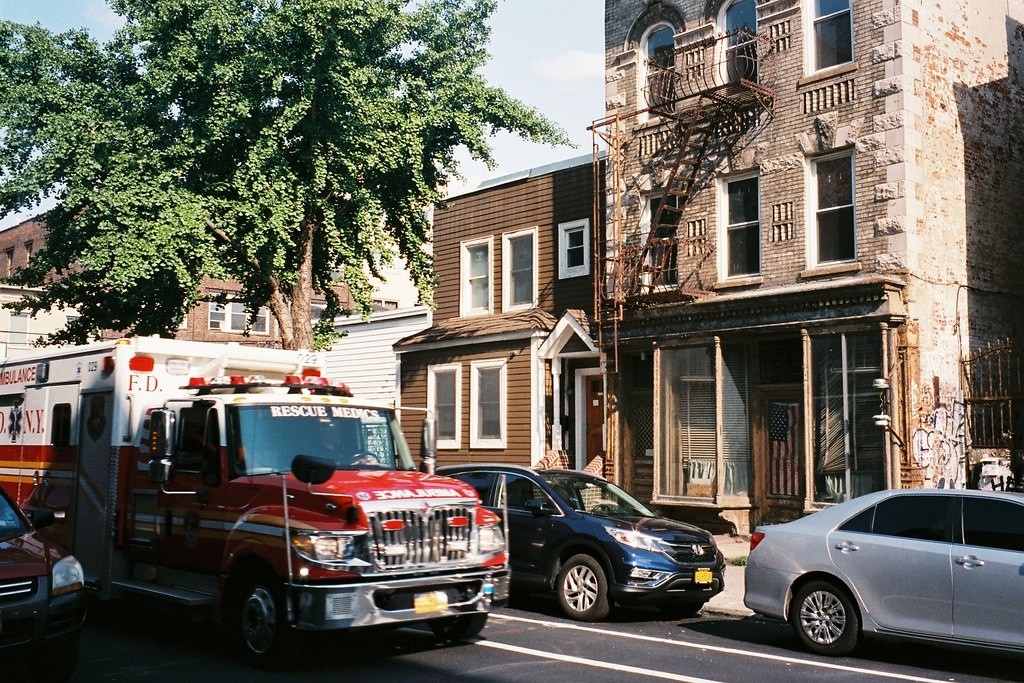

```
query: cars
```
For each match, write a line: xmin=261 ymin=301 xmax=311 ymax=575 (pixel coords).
xmin=741 ymin=487 xmax=1024 ymax=657
xmin=0 ymin=484 xmax=90 ymax=680
xmin=433 ymin=463 xmax=727 ymax=622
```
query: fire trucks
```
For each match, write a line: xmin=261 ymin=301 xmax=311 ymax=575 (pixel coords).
xmin=0 ymin=333 xmax=511 ymax=673
